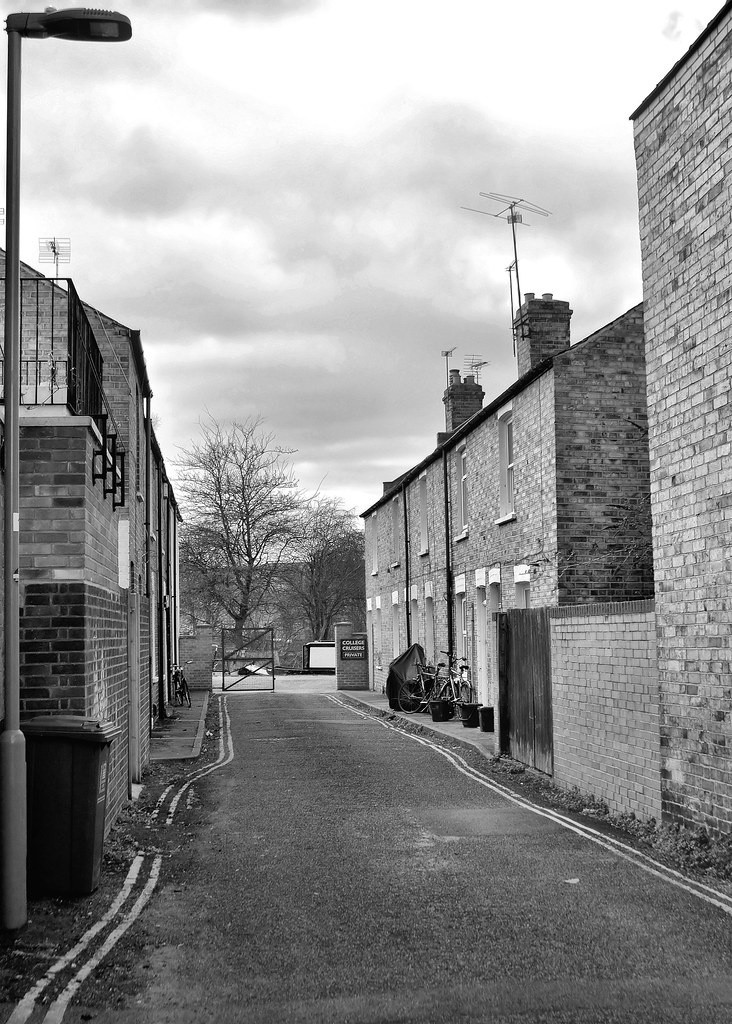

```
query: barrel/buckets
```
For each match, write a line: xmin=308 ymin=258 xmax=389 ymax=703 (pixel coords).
xmin=430 ymin=701 xmax=451 ymax=722
xmin=460 ymin=703 xmax=483 ymax=728
xmin=478 ymin=707 xmax=494 ymax=732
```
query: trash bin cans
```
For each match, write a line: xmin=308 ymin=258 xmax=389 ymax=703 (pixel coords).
xmin=0 ymin=714 xmax=113 ymax=902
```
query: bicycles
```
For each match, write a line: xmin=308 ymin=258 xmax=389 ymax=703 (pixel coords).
xmin=170 ymin=660 xmax=194 ymax=708
xmin=397 ymin=658 xmax=450 ymax=713
xmin=437 ymin=650 xmax=472 ymax=719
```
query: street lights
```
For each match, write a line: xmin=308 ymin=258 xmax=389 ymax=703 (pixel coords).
xmin=0 ymin=6 xmax=132 ymax=931
